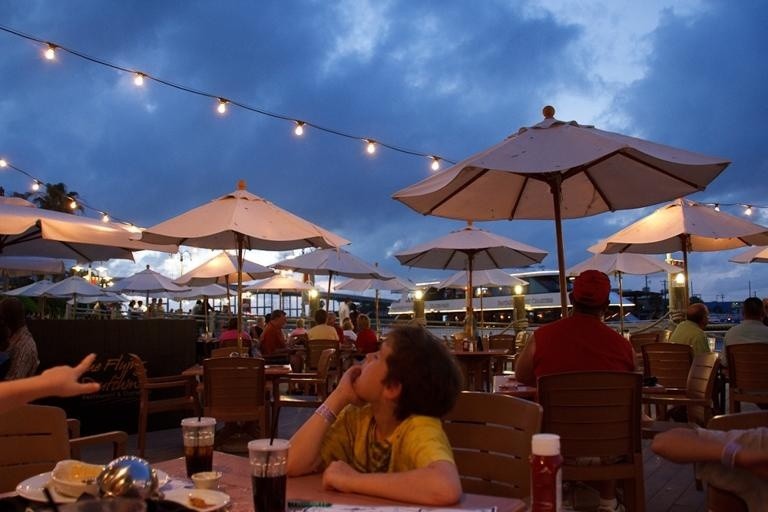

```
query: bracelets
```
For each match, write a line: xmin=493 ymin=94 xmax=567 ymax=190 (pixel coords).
xmin=718 ymin=441 xmax=742 ymax=468
xmin=314 ymin=403 xmax=336 ymax=424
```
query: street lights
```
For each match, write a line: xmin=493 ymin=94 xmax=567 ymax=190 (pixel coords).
xmin=511 ymin=286 xmax=527 ymax=322
xmin=659 ymin=279 xmax=668 ymax=309
xmin=169 ymin=250 xmax=193 ymax=310
xmin=671 ymin=274 xmax=686 ymax=315
xmin=71 ymin=254 xmax=115 ymax=289
xmin=643 ymin=275 xmax=652 ymax=292
xmin=243 ymin=291 xmax=251 ymax=315
xmin=689 ymin=280 xmax=695 ymax=297
xmin=308 ymin=289 xmax=318 ymax=320
xmin=412 ymin=290 xmax=424 ymax=324
xmin=222 ymin=298 xmax=231 ymax=313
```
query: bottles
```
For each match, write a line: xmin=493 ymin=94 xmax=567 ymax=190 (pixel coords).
xmin=468 ymin=335 xmax=478 ymax=353
xmin=229 ymin=352 xmax=248 ymax=358
xmin=482 ymin=336 xmax=489 ymax=352
xmin=527 ymin=433 xmax=564 ymax=512
xmin=462 ymin=337 xmax=469 ymax=351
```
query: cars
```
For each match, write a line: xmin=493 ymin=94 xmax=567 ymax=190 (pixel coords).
xmin=709 ymin=314 xmax=740 ymax=323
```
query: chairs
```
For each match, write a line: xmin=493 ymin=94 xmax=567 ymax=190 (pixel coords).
xmin=132 ymin=323 xmax=768 ymax=511
xmin=0 ymin=405 xmax=71 ymax=495
xmin=63 ymin=418 xmax=128 ymax=465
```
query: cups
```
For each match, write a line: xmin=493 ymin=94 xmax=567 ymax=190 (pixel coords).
xmin=248 ymin=438 xmax=290 ymax=511
xmin=181 ymin=416 xmax=216 ymax=478
xmin=189 ymin=469 xmax=222 ymax=487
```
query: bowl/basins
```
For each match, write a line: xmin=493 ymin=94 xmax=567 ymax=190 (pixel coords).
xmin=51 ymin=459 xmax=105 ymax=497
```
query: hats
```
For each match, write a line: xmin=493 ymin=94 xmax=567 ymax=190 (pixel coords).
xmin=574 ymin=270 xmax=610 ymax=306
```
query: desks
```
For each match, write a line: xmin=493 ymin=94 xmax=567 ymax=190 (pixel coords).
xmin=0 ymin=445 xmax=528 ymax=512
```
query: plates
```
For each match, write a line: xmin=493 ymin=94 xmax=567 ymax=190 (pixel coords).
xmin=164 ymin=488 xmax=231 ymax=511
xmin=15 ymin=465 xmax=169 ymax=505
xmin=498 ymin=383 xmax=518 ymax=388
xmin=503 ymin=376 xmax=516 ymax=380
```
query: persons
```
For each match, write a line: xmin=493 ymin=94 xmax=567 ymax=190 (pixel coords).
xmin=762 ymin=298 xmax=767 ymax=327
xmin=286 ymin=320 xmax=462 ymax=505
xmin=91 ymin=294 xmax=378 ymax=396
xmin=717 ymin=297 xmax=767 ymax=368
xmin=513 ymin=270 xmax=638 ymax=390
xmin=650 ymin=426 xmax=767 ymax=478
xmin=668 ymin=303 xmax=711 ymax=355
xmin=0 ymin=352 xmax=100 ymax=415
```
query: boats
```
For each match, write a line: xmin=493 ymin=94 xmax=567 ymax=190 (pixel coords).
xmin=388 ymin=271 xmax=636 ymax=327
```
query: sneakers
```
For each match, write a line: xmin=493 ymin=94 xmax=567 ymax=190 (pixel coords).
xmin=598 ymin=503 xmax=625 ymax=512
